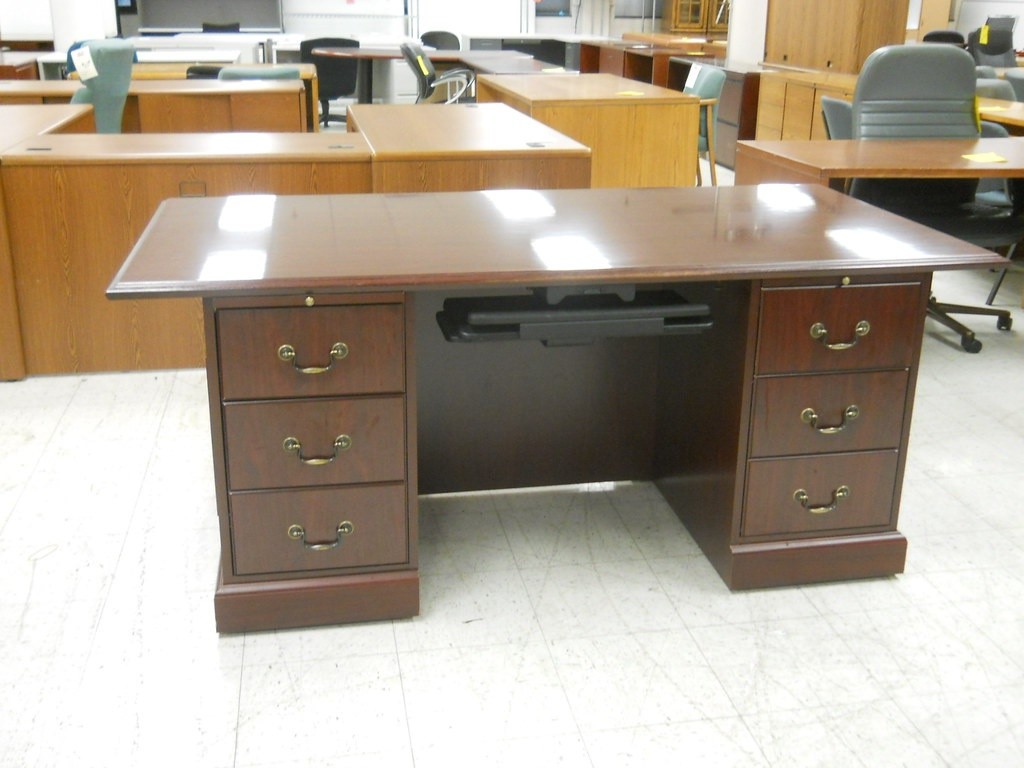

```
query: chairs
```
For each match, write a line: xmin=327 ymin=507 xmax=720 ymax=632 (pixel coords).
xmin=420 ymin=31 xmax=460 ymax=50
xmin=69 ymin=40 xmax=134 ymax=134
xmin=67 ymin=38 xmax=138 ymax=75
xmin=202 ymin=23 xmax=240 ymax=33
xmin=300 ymin=38 xmax=359 ymax=128
xmin=679 ymin=62 xmax=726 ymax=187
xmin=186 ymin=66 xmax=223 ymax=79
xmin=218 ymin=66 xmax=299 ymax=80
xmin=820 ymin=14 xmax=1024 ymax=351
xmin=400 ymin=42 xmax=476 ymax=104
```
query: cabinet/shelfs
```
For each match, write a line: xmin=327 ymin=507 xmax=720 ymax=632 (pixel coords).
xmin=470 ymin=0 xmax=910 ymax=171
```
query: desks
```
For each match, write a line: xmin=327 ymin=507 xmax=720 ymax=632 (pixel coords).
xmin=734 ymin=57 xmax=1024 ymax=189
xmin=106 ymin=180 xmax=1008 ymax=635
xmin=0 ymin=33 xmax=703 ymax=384
